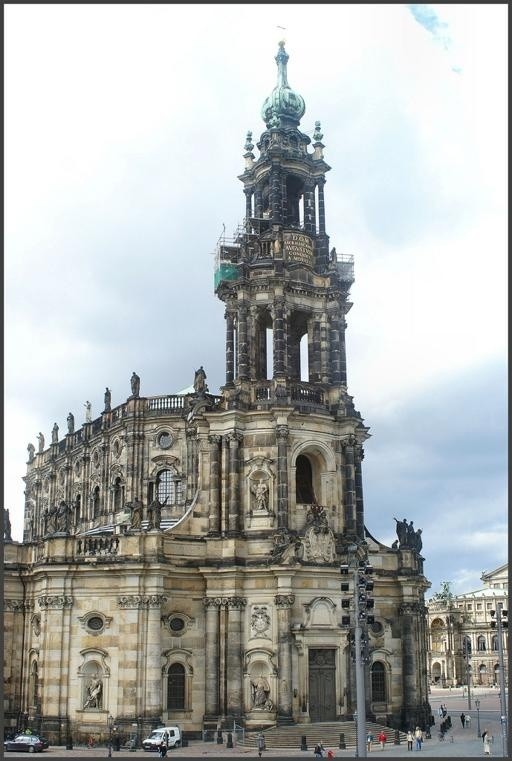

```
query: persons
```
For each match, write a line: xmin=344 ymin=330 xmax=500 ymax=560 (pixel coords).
xmin=315 ymin=739 xmax=325 ymax=759
xmin=130 ymin=372 xmax=141 ymax=397
xmin=460 ymin=713 xmax=465 ymax=729
xmin=393 ymin=517 xmax=408 ymax=545
xmin=481 ymin=728 xmax=488 ymax=743
xmin=258 ymin=730 xmax=266 ymax=752
xmin=82 ymin=673 xmax=103 ymax=711
xmin=162 ymin=742 xmax=169 ymax=761
xmin=414 ymin=727 xmax=424 ymax=750
xmin=252 ymin=477 xmax=270 ymax=511
xmin=406 ymin=521 xmax=414 ymax=548
xmin=367 ymin=728 xmax=374 ymax=753
xmin=407 ymin=731 xmax=414 ymax=752
xmin=442 ymin=704 xmax=447 ymax=719
xmin=27 ymin=443 xmax=36 ymax=462
xmin=329 ymin=246 xmax=338 ymax=265
xmin=5 ymin=728 xmax=40 ymax=742
xmin=83 ymin=400 xmax=93 ymax=424
xmin=438 ymin=705 xmax=443 ymax=719
xmin=147 ymin=495 xmax=170 ymax=528
xmin=56 ymin=500 xmax=70 ymax=531
xmin=51 ymin=422 xmax=60 ymax=444
xmin=415 ymin=527 xmax=423 ymax=553
xmin=483 ymin=731 xmax=493 ymax=756
xmin=124 ymin=496 xmax=143 ymax=531
xmin=158 ymin=742 xmax=164 ymax=760
xmin=249 ymin=673 xmax=271 ymax=710
xmin=193 ymin=365 xmax=208 ymax=394
xmin=67 ymin=413 xmax=75 ymax=435
xmin=378 ymin=731 xmax=387 ymax=753
xmin=44 ymin=505 xmax=58 ymax=536
xmin=464 ymin=713 xmax=471 ymax=728
xmin=36 ymin=432 xmax=45 ymax=454
xmin=104 ymin=387 xmax=112 ymax=411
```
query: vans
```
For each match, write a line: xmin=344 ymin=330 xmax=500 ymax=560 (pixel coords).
xmin=142 ymin=726 xmax=181 ymax=750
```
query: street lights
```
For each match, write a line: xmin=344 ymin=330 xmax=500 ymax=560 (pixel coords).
xmin=475 ymin=698 xmax=481 ymax=737
xmin=107 ymin=715 xmax=113 ymax=758
xmin=339 ymin=559 xmax=375 ymax=758
xmin=490 ymin=604 xmax=510 ymax=758
xmin=463 ymin=638 xmax=473 ymax=710
xmin=353 ymin=711 xmax=359 ymax=759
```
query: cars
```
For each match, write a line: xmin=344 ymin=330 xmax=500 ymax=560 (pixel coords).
xmin=3 ymin=734 xmax=50 ymax=753
